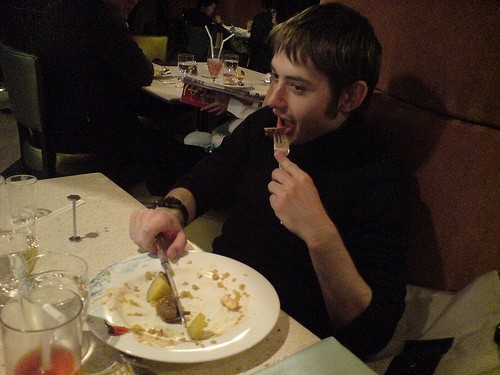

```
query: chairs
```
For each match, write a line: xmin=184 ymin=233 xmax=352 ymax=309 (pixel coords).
xmin=0 ymin=44 xmax=129 ymax=178
xmin=186 ymin=25 xmax=207 ymax=60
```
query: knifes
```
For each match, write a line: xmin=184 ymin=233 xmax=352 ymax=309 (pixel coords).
xmin=155 ymin=236 xmax=186 ymax=325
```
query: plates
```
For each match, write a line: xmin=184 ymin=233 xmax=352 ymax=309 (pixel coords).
xmin=86 ymin=250 xmax=280 ymax=363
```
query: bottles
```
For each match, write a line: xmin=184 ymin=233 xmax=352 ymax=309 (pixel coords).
xmin=247 ymin=20 xmax=251 ymax=33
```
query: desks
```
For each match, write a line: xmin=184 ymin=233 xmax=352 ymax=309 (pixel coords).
xmin=141 ymin=61 xmax=270 ymax=192
xmin=0 ymin=172 xmax=321 ymax=375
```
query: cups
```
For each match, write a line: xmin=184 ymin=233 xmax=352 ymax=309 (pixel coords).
xmin=224 ymin=54 xmax=239 ymax=71
xmin=0 ymin=286 xmax=83 ymax=375
xmin=6 ymin=174 xmax=38 ymax=226
xmin=15 ymin=252 xmax=89 ymax=324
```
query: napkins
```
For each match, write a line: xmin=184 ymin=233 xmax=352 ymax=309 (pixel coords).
xmin=250 ymin=336 xmax=378 ymax=375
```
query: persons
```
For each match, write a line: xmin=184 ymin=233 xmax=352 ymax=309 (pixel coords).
xmin=38 ymin=0 xmax=154 ymax=188
xmin=129 ymin=2 xmax=406 ymax=361
xmin=182 ymin=0 xmax=230 ymax=40
xmin=240 ymin=0 xmax=288 ymax=68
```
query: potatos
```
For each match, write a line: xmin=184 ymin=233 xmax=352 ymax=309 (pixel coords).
xmin=187 ymin=313 xmax=207 ymax=340
xmin=147 ymin=274 xmax=172 ymax=302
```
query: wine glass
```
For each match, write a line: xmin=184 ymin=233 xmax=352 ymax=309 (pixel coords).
xmin=178 ymin=53 xmax=194 ymax=82
xmin=207 ymin=46 xmax=224 ymax=83
xmin=223 ymin=67 xmax=246 ymax=85
xmin=0 ymin=209 xmax=39 ymax=298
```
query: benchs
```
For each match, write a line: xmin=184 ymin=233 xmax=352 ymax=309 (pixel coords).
xmin=323 ymin=0 xmax=500 ymax=375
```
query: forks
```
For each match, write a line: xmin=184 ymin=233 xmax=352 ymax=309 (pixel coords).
xmin=273 ymin=133 xmax=290 ymax=224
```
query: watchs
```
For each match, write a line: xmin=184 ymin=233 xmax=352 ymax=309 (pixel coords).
xmin=153 ymin=196 xmax=188 ymax=227
